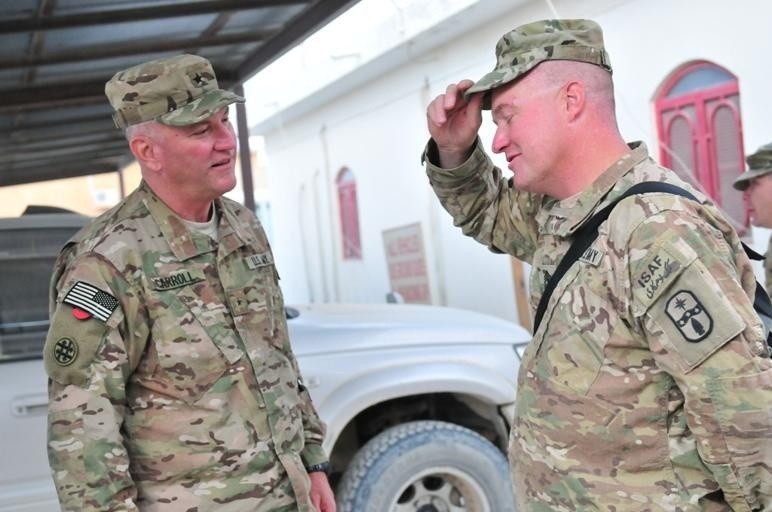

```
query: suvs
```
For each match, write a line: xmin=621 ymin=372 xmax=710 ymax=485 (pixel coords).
xmin=1 ymin=215 xmax=537 ymax=511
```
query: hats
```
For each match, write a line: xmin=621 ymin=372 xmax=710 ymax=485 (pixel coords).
xmin=104 ymin=52 xmax=247 ymax=128
xmin=732 ymin=141 xmax=772 ymax=193
xmin=463 ymin=19 xmax=613 ymax=110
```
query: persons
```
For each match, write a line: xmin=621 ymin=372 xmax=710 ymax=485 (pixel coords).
xmin=421 ymin=15 xmax=772 ymax=510
xmin=40 ymin=52 xmax=340 ymax=512
xmin=732 ymin=140 xmax=772 ymax=303
